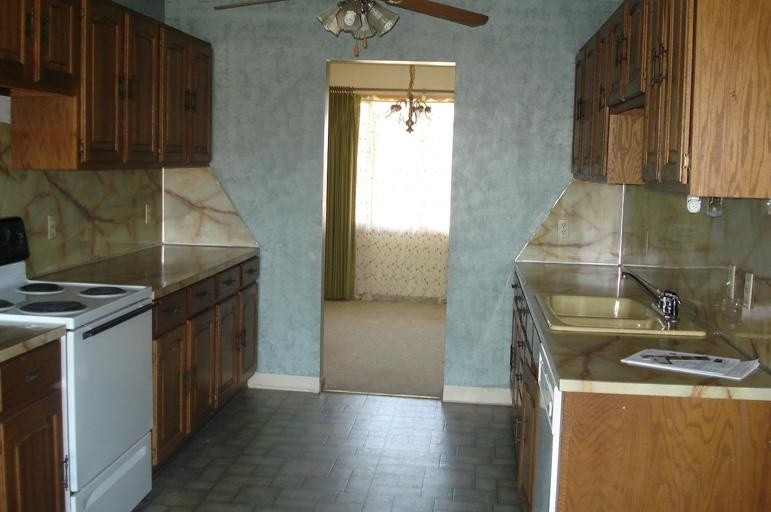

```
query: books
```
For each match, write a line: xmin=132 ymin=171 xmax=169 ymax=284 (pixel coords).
xmin=619 ymin=346 xmax=761 ymax=381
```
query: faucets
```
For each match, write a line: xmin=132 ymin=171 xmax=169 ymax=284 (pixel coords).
xmin=619 ymin=270 xmax=683 ymax=321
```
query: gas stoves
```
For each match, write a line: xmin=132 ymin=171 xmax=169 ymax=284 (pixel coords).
xmin=0 ymin=281 xmax=153 ymax=319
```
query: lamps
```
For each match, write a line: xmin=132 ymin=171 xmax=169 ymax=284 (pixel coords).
xmin=315 ymin=0 xmax=399 ymax=57
xmin=386 ymin=65 xmax=432 ymax=134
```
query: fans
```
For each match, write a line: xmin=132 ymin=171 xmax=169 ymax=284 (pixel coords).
xmin=213 ymin=0 xmax=489 ymax=28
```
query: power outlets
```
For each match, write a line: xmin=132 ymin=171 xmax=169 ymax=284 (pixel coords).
xmin=557 ymin=220 xmax=568 ymax=239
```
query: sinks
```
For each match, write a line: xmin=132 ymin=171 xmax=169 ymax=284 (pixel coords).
xmin=547 ymin=290 xmax=657 ymax=317
xmin=554 ymin=314 xmax=665 ymax=334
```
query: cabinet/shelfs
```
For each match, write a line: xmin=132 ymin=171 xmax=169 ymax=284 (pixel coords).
xmin=569 ymin=0 xmax=771 ymax=200
xmin=0 ymin=0 xmax=213 ymax=171
xmin=508 ymin=273 xmax=771 ymax=512
xmin=152 ymin=256 xmax=260 ymax=477
xmin=0 ymin=338 xmax=66 ymax=512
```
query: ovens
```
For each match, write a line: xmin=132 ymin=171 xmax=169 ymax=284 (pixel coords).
xmin=60 ymin=300 xmax=154 ymax=512
